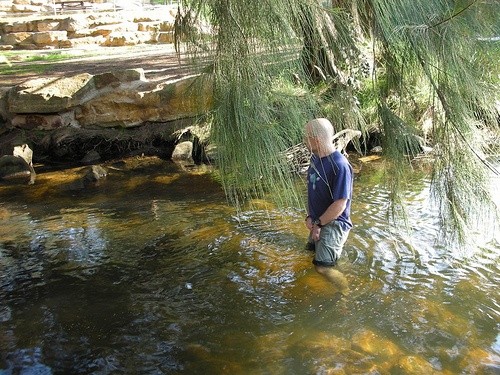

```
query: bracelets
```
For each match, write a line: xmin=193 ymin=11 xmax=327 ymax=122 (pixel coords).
xmin=305 ymin=214 xmax=311 ymax=222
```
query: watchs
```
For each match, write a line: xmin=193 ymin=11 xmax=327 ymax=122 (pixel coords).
xmin=314 ymin=218 xmax=326 ymax=229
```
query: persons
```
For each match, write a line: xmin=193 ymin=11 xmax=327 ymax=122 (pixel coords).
xmin=302 ymin=118 xmax=356 ymax=298
